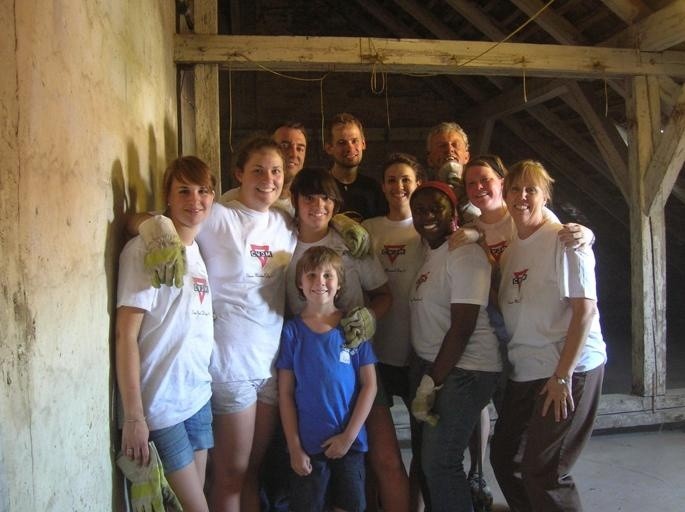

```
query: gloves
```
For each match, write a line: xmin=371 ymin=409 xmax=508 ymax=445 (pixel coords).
xmin=138 ymin=215 xmax=187 ymax=288
xmin=117 ymin=442 xmax=184 ymax=512
xmin=332 ymin=214 xmax=371 ymax=258
xmin=340 ymin=304 xmax=376 ymax=348
xmin=409 ymin=376 xmax=444 ymax=426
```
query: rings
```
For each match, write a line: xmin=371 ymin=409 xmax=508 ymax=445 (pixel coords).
xmin=126 ymin=446 xmax=134 ymax=458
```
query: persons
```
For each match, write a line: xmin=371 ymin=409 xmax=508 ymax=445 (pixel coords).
xmin=220 ymin=120 xmax=309 ymax=218
xmin=274 ymin=243 xmax=384 ymax=512
xmin=423 ymin=120 xmax=496 ymax=502
xmin=283 ymin=169 xmax=414 ymax=511
xmin=359 ymin=152 xmax=487 ymax=512
xmin=462 ymin=148 xmax=596 ymax=512
xmin=488 ymin=158 xmax=608 ymax=512
xmin=405 ymin=178 xmax=509 ymax=512
xmin=125 ymin=135 xmax=373 ymax=511
xmin=115 ymin=155 xmax=218 ymax=511
xmin=322 ymin=109 xmax=393 ymax=217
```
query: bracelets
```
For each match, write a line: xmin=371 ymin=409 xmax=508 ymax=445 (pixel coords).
xmin=122 ymin=415 xmax=151 ymax=423
xmin=551 ymin=371 xmax=572 ymax=386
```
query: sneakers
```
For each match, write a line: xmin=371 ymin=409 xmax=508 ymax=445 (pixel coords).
xmin=469 ymin=476 xmax=493 ymax=507
